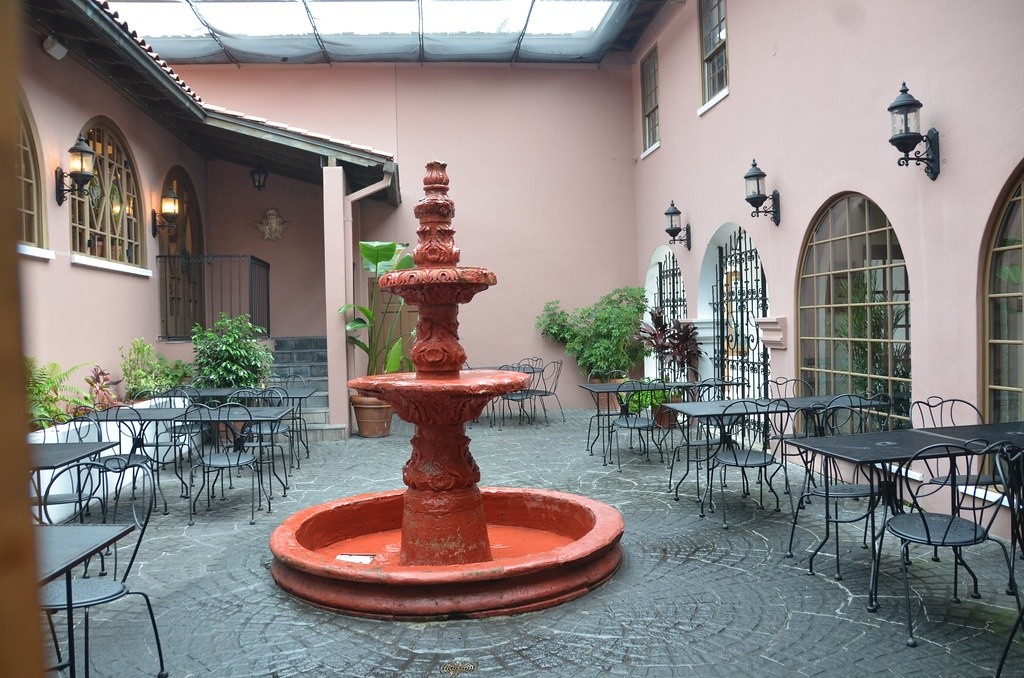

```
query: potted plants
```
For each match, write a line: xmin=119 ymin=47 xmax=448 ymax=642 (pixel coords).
xmin=535 ymin=285 xmax=709 ymax=428
xmin=337 ymin=241 xmax=418 ymax=437
xmin=191 ymin=312 xmax=275 ymax=446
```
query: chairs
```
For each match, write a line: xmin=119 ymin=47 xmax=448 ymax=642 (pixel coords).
xmin=585 ymin=366 xmax=1024 ymax=678
xmin=27 ymin=372 xmax=310 ymax=678
xmin=462 ymin=357 xmax=566 ymax=431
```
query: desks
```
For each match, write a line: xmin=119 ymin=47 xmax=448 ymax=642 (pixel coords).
xmin=662 ymin=399 xmax=796 ymax=517
xmin=32 ymin=524 xmax=136 ymax=678
xmin=913 ymin=421 xmax=1024 ymax=596
xmin=667 ymin=382 xmax=748 ymax=401
xmin=760 ymin=394 xmax=888 ymax=485
xmin=228 ymin=389 xmax=318 ymax=467
xmin=173 ymin=406 xmax=294 ymax=514
xmin=150 ymin=389 xmax=239 ymax=408
xmin=579 ymin=382 xmax=671 ymax=463
xmin=464 ymin=367 xmax=544 ymax=373
xmin=73 ymin=409 xmax=190 ymax=515
xmin=27 ymin=442 xmax=121 ymax=524
xmin=784 ymin=430 xmax=1001 ymax=612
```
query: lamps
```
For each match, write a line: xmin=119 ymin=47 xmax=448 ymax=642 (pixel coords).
xmin=56 ymin=133 xmax=95 ymax=207
xmin=151 ymin=183 xmax=180 ymax=237
xmin=665 ymin=200 xmax=691 ymax=251
xmin=250 ymin=161 xmax=267 ymax=191
xmin=43 ymin=35 xmax=68 ymax=61
xmin=744 ymin=158 xmax=781 ymax=226
xmin=887 ymin=81 xmax=941 ymax=181
